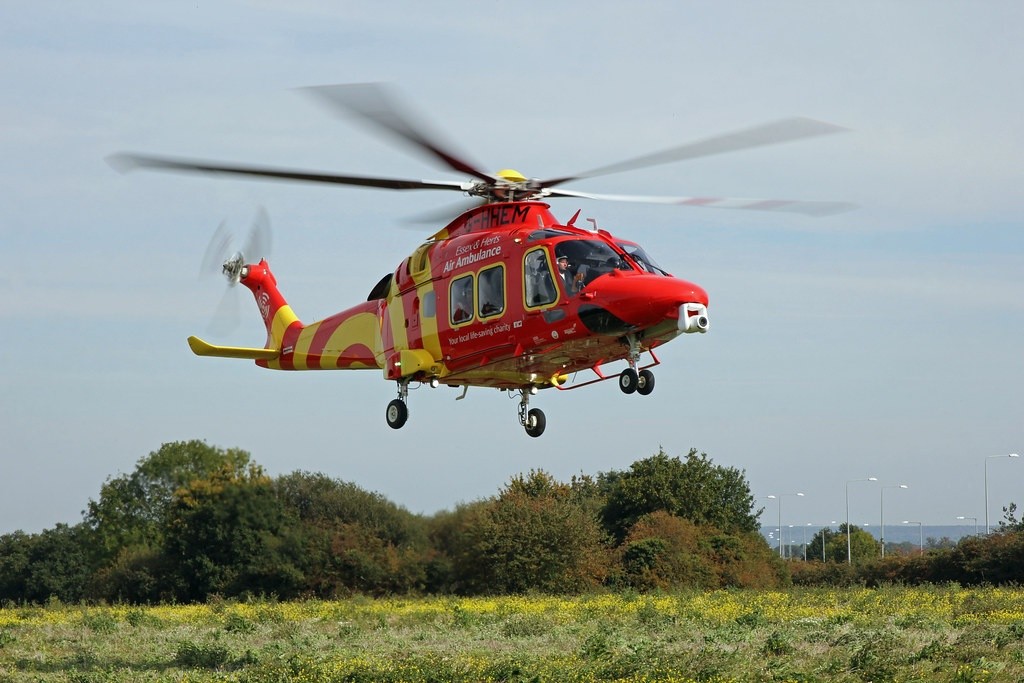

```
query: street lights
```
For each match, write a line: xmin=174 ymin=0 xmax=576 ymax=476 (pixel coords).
xmin=788 ymin=525 xmax=794 ymax=558
xmin=802 ymin=522 xmax=813 ymax=562
xmin=955 ymin=516 xmax=978 ymax=536
xmin=984 ymin=453 xmax=1020 ymax=534
xmin=902 ymin=520 xmax=923 ymax=556
xmin=880 ymin=484 xmax=909 ymax=558
xmin=845 ymin=478 xmax=879 ymax=563
xmin=823 ymin=521 xmax=838 ymax=563
xmin=778 ymin=493 xmax=805 ymax=556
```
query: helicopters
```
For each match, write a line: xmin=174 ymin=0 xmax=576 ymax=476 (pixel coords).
xmin=111 ymin=83 xmax=877 ymax=440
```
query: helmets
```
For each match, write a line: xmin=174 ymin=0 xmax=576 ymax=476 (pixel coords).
xmin=556 ymin=252 xmax=568 ymax=265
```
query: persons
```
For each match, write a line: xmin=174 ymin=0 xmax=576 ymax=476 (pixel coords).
xmin=545 ymin=252 xmax=584 ymax=302
